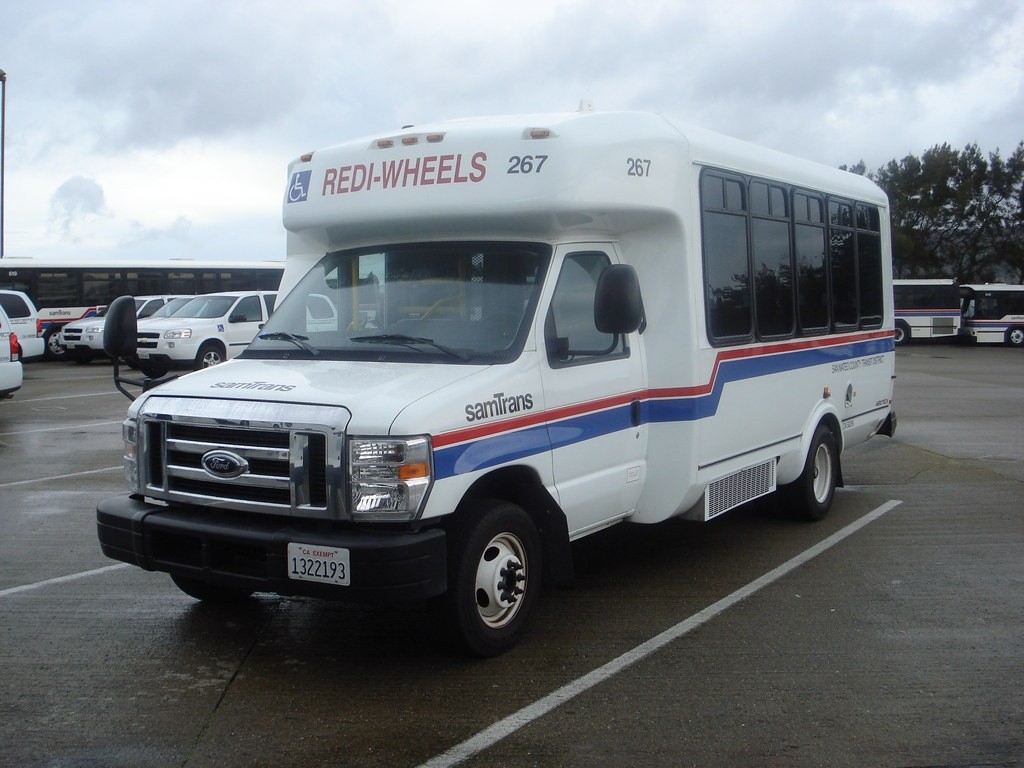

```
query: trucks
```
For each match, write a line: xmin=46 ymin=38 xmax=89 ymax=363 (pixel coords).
xmin=94 ymin=108 xmax=895 ymax=654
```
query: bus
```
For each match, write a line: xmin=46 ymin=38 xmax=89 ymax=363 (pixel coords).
xmin=893 ymin=277 xmax=964 ymax=344
xmin=960 ymin=280 xmax=1024 ymax=347
xmin=0 ymin=257 xmax=286 ymax=361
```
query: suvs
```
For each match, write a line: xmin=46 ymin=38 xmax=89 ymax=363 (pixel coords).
xmin=0 ymin=289 xmax=45 ymax=362
xmin=137 ymin=290 xmax=338 ymax=378
xmin=59 ymin=295 xmax=179 ymax=363
xmin=0 ymin=301 xmax=24 ymax=400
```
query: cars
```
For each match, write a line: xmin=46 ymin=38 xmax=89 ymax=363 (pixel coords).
xmin=126 ymin=295 xmax=196 ymax=368
